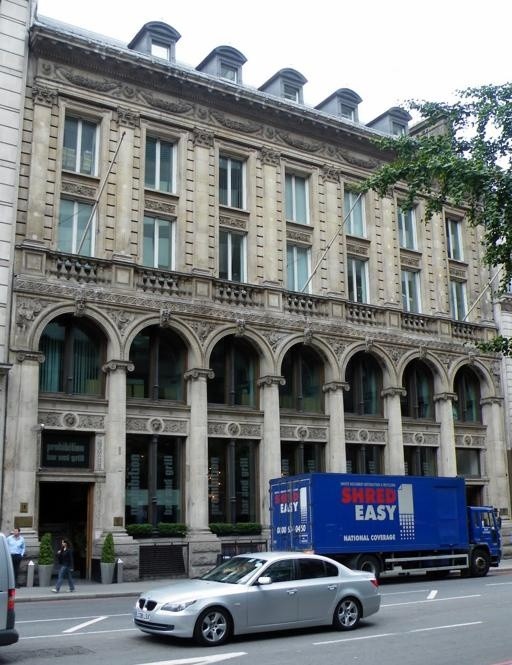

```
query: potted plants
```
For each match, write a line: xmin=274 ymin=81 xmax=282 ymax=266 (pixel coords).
xmin=38 ymin=533 xmax=54 ymax=587
xmin=100 ymin=533 xmax=116 ymax=585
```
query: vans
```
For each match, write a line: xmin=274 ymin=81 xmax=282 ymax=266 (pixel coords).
xmin=1 ymin=533 xmax=20 ymax=646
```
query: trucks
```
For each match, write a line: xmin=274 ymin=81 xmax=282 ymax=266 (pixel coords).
xmin=266 ymin=472 xmax=503 ymax=580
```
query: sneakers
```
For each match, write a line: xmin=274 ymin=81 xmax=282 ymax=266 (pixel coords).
xmin=52 ymin=588 xmax=74 ymax=593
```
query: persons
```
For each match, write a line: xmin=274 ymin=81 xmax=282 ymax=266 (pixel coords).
xmin=50 ymin=537 xmax=75 ymax=593
xmin=5 ymin=526 xmax=26 ymax=588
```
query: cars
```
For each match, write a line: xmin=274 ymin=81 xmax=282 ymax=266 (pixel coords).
xmin=132 ymin=549 xmax=383 ymax=647
